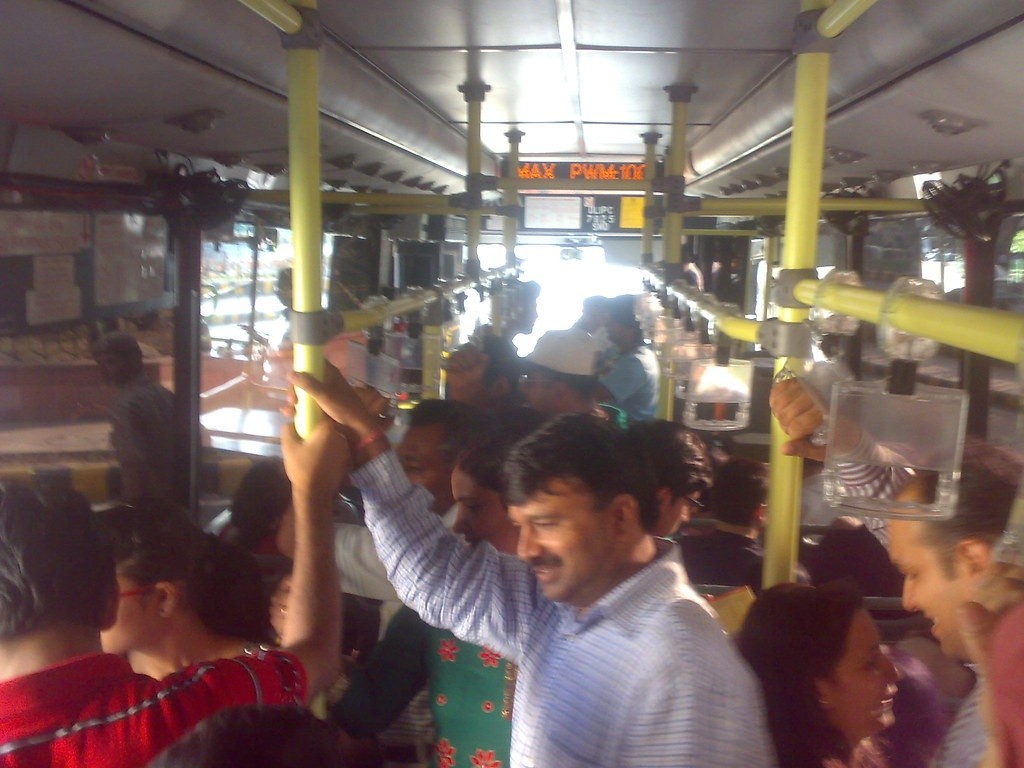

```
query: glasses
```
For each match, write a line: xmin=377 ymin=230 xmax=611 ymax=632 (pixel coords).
xmin=519 ymin=373 xmax=559 ymax=389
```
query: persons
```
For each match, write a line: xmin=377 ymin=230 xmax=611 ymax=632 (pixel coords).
xmin=0 ymin=267 xmax=1024 ymax=768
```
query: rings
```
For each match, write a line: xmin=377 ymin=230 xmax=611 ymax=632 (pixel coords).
xmin=281 ymin=605 xmax=287 ymax=615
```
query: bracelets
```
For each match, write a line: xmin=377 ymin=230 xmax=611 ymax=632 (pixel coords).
xmin=351 ymin=428 xmax=384 ymax=451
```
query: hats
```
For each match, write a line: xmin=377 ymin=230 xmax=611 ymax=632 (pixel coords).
xmin=526 ymin=330 xmax=597 ymax=375
xmin=89 ymin=332 xmax=139 ymax=356
